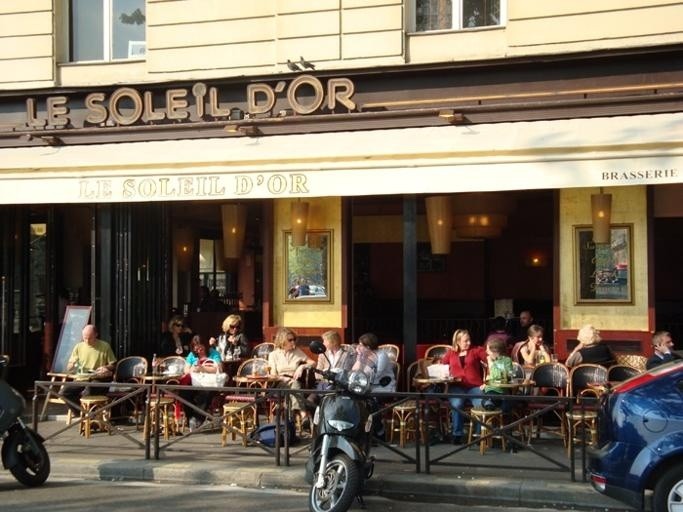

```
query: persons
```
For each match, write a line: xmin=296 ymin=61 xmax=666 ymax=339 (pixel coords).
xmin=288 ymin=278 xmax=326 ymax=299
xmin=57 ymin=324 xmax=117 ymax=420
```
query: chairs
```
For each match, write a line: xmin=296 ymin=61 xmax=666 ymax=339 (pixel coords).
xmin=378 ymin=344 xmax=647 ymax=458
xmin=340 ymin=345 xmax=356 ymax=359
xmin=78 ymin=344 xmax=314 ymax=455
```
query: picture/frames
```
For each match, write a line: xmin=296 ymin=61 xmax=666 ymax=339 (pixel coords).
xmin=572 ymin=223 xmax=636 ymax=307
xmin=281 ymin=228 xmax=334 ymax=305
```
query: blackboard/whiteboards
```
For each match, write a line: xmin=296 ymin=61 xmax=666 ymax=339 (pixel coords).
xmin=49 ymin=305 xmax=93 ymax=373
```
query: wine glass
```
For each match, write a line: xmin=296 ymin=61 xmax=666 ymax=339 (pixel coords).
xmin=264 ymin=361 xmax=272 ymax=377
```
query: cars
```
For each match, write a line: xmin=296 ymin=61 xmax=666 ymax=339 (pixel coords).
xmin=287 ymin=284 xmax=326 ymax=299
xmin=0 ymin=351 xmax=52 ymax=491
xmin=587 ymin=235 xmax=628 ymax=289
xmin=13 ymin=289 xmax=19 ymax=332
xmin=29 ymin=293 xmax=44 ymax=330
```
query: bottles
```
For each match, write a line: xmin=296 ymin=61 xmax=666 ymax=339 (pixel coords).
xmin=151 ymin=353 xmax=158 ymax=375
xmin=252 ymin=355 xmax=259 ymax=377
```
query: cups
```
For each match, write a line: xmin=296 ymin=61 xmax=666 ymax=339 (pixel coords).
xmin=441 ymin=368 xmax=450 ymax=381
xmin=494 ymin=367 xmax=518 ymax=385
xmin=216 ymin=346 xmax=242 ymax=361
xmin=535 ymin=350 xmax=559 ymax=367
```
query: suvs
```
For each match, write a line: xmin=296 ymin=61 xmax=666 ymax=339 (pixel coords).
xmin=583 ymin=357 xmax=683 ymax=511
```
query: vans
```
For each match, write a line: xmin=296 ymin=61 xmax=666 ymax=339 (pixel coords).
xmin=199 ymin=269 xmax=227 ymax=297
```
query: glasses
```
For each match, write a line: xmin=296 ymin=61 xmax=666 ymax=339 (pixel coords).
xmin=230 ymin=326 xmax=240 ymax=330
xmin=288 ymin=339 xmax=296 ymax=342
xmin=175 ymin=324 xmax=184 ymax=328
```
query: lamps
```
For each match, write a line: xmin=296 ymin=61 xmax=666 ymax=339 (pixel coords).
xmin=221 ymin=200 xmax=246 ymax=258
xmin=290 ymin=197 xmax=308 ymax=246
xmin=438 ymin=110 xmax=461 ymax=123
xmin=589 ymin=187 xmax=612 ymax=244
xmin=41 ymin=136 xmax=60 ymax=145
xmin=223 ymin=126 xmax=256 ymax=135
xmin=425 ymin=192 xmax=453 ymax=256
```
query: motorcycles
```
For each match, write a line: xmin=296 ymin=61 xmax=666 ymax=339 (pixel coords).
xmin=303 ymin=341 xmax=393 ymax=511
